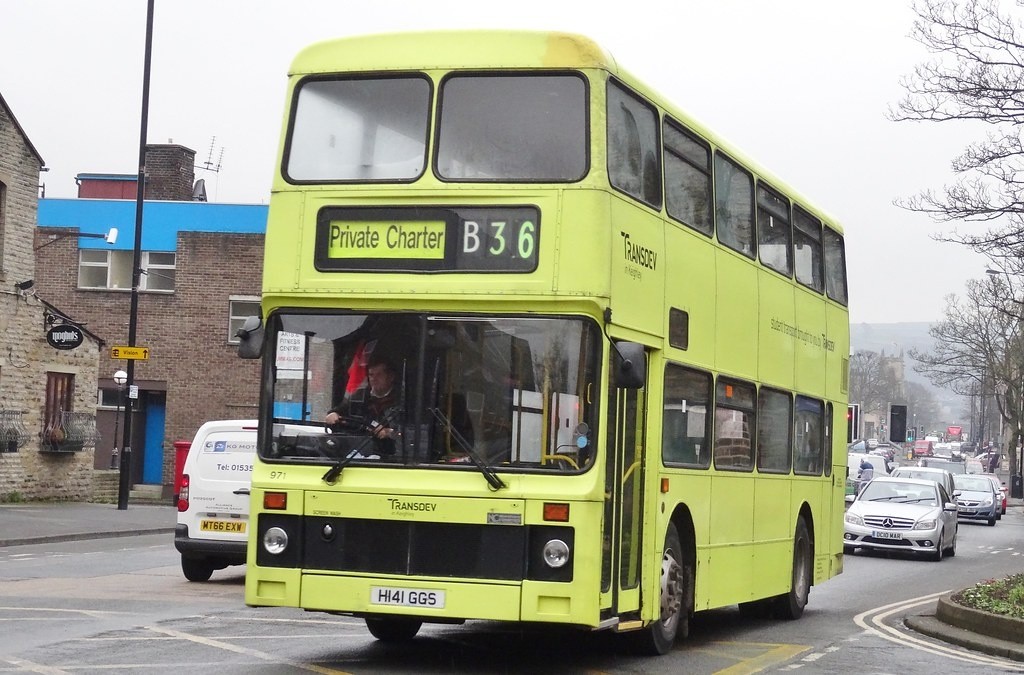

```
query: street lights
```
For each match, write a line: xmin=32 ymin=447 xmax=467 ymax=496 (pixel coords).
xmin=110 ymin=368 xmax=131 ymax=471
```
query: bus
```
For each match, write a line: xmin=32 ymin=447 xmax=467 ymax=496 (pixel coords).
xmin=255 ymin=40 xmax=849 ymax=659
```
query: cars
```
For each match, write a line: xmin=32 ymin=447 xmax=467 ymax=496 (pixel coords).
xmin=856 ymin=454 xmax=892 ymax=473
xmin=980 ymin=473 xmax=1009 ymax=514
xmin=913 ymin=426 xmax=943 ymax=443
xmin=991 ymin=478 xmax=1003 ymax=520
xmin=914 ymin=442 xmax=1001 ymax=474
xmin=844 ymin=478 xmax=960 ymax=559
xmin=955 ymin=476 xmax=997 ymax=522
xmin=866 ymin=436 xmax=895 ymax=462
xmin=847 ymin=459 xmax=874 ymax=495
xmin=890 ymin=465 xmax=959 ymax=533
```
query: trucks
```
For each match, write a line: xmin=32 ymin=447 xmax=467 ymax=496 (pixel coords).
xmin=944 ymin=426 xmax=962 ymax=442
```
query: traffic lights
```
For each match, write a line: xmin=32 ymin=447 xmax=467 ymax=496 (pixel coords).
xmin=881 ymin=424 xmax=883 ymax=432
xmin=848 ymin=405 xmax=853 ymax=443
xmin=911 ymin=429 xmax=915 ymax=441
xmin=907 ymin=430 xmax=912 ymax=441
xmin=914 ymin=427 xmax=917 ymax=437
xmin=920 ymin=425 xmax=924 ymax=433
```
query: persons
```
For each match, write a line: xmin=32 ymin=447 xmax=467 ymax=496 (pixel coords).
xmin=326 ymin=356 xmax=400 ymax=440
xmin=858 ymin=459 xmax=874 ymax=481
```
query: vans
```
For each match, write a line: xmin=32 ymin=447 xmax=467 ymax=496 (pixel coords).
xmin=913 ymin=440 xmax=932 ymax=457
xmin=173 ymin=418 xmax=336 ymax=585
